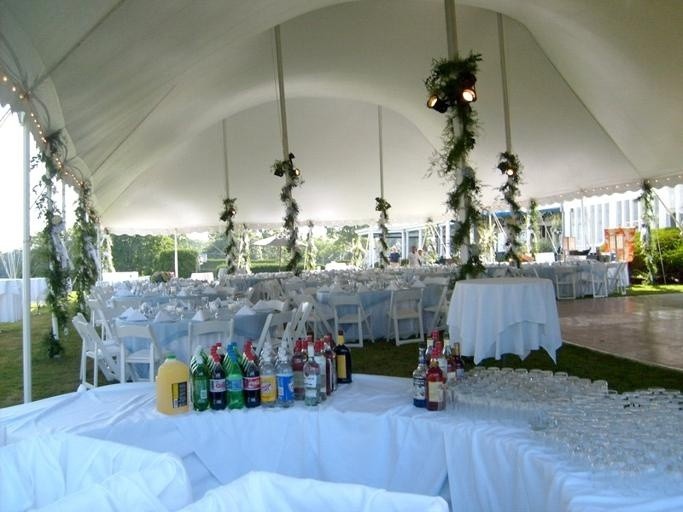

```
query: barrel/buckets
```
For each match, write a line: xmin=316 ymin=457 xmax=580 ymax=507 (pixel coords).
xmin=157 ymin=354 xmax=191 ymax=415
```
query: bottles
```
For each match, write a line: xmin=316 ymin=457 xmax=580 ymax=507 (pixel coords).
xmin=411 ymin=334 xmax=464 ymax=412
xmin=189 ymin=328 xmax=353 ymax=412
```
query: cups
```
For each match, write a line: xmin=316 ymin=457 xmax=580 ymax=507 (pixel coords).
xmin=441 ymin=364 xmax=608 ymax=422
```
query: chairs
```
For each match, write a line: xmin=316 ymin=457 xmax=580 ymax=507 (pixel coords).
xmin=71 ymin=260 xmax=628 ymax=390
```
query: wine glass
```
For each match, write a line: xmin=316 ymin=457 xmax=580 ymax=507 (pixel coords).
xmin=300 ymin=264 xmax=457 ymax=289
xmin=541 ymin=385 xmax=683 ymax=496
xmin=122 ymin=278 xmax=242 ymax=321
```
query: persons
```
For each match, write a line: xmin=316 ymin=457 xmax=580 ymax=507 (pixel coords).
xmin=389 ymin=245 xmax=422 ymax=266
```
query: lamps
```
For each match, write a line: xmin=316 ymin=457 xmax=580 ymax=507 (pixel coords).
xmin=497 ymin=161 xmax=515 ymax=177
xmin=425 ymin=94 xmax=449 ymax=115
xmin=375 ymin=202 xmax=389 ymax=212
xmin=456 ymin=87 xmax=477 ymax=107
xmin=221 ymin=207 xmax=236 ymax=222
xmin=273 ymin=167 xmax=301 ymax=180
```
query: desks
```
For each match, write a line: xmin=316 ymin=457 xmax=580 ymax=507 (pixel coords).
xmin=0 ymin=373 xmax=683 ymax=512
